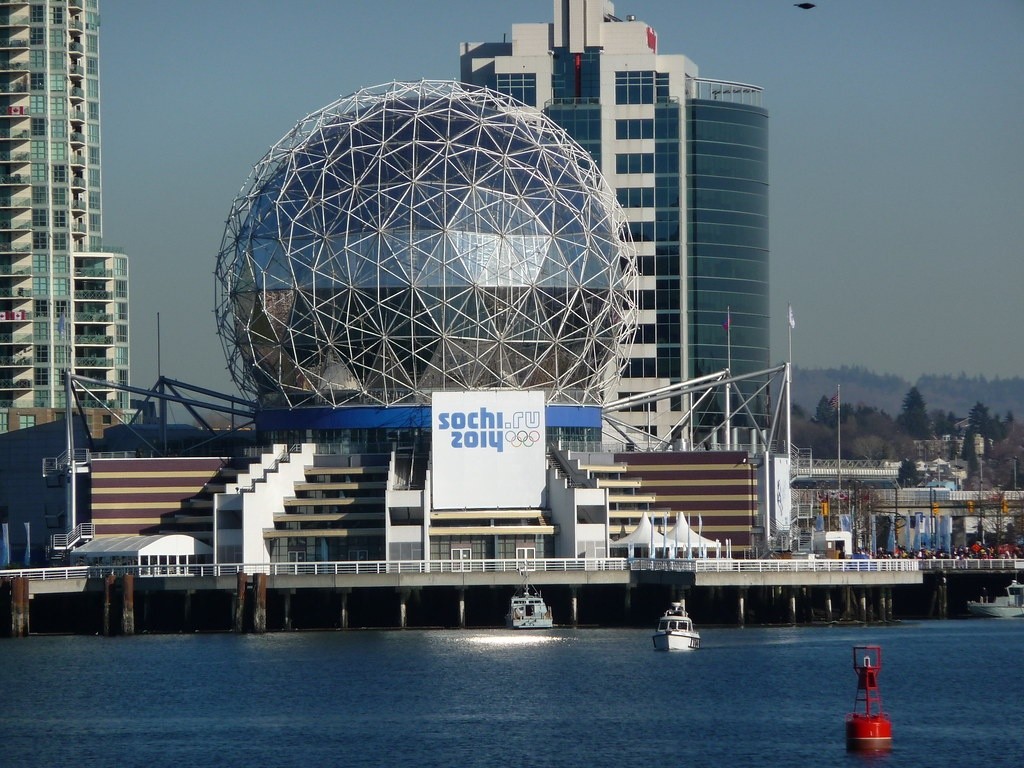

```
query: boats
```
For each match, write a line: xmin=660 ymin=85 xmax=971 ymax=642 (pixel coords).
xmin=652 ymin=602 xmax=701 ymax=653
xmin=505 ymin=566 xmax=554 ymax=628
xmin=967 ymin=579 xmax=1024 ymax=618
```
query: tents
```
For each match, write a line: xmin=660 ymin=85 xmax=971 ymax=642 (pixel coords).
xmin=609 ymin=512 xmax=722 ymax=559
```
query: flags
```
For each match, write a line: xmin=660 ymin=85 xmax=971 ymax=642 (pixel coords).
xmin=723 ymin=317 xmax=731 ymax=331
xmin=788 ymin=304 xmax=796 ymax=329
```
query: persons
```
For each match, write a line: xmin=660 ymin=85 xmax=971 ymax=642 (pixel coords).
xmin=839 ymin=541 xmax=1024 ymax=559
